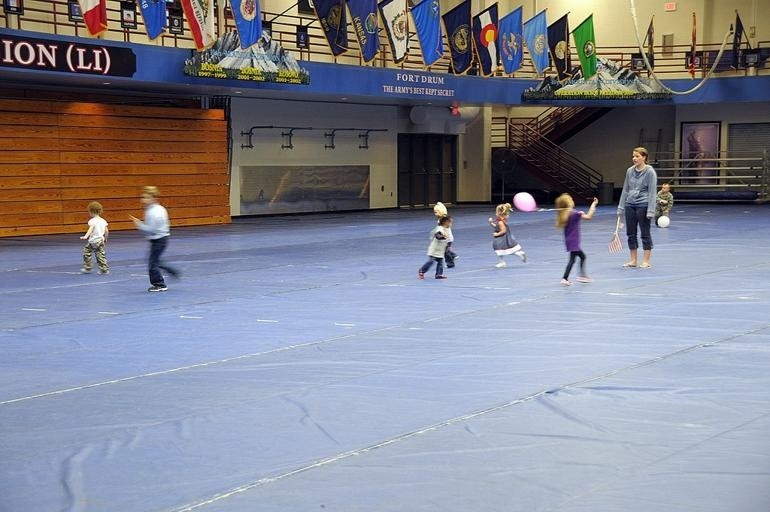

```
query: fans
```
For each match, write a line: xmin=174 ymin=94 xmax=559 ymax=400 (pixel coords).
xmin=491 ymin=147 xmax=516 ymax=206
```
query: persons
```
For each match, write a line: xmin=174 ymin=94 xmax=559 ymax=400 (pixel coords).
xmin=489 ymin=203 xmax=527 ymax=267
xmin=418 ymin=213 xmax=453 ymax=279
xmin=79 ymin=201 xmax=111 ymax=275
xmin=127 ymin=185 xmax=183 ymax=292
xmin=655 ymin=183 xmax=674 ymax=227
xmin=616 ymin=147 xmax=657 ymax=268
xmin=434 ymin=201 xmax=460 ymax=269
xmin=556 ymin=193 xmax=599 ymax=286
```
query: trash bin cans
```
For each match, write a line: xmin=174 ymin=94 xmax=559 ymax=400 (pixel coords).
xmin=597 ymin=182 xmax=615 ymax=205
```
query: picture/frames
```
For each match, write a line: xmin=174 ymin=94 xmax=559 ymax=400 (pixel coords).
xmin=679 ymin=120 xmax=722 ymax=185
xmin=298 ymin=0 xmax=316 ymax=15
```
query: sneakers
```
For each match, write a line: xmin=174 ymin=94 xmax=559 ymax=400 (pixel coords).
xmin=419 ymin=270 xmax=424 ymax=278
xmin=560 ymin=279 xmax=573 ymax=286
xmin=80 ymin=268 xmax=93 ymax=274
xmin=520 ymin=251 xmax=526 ymax=263
xmin=97 ymin=271 xmax=109 ymax=274
xmin=148 ymin=286 xmax=168 ymax=292
xmin=640 ymin=264 xmax=651 ymax=269
xmin=435 ymin=275 xmax=446 ymax=279
xmin=496 ymin=262 xmax=507 ymax=267
xmin=576 ymin=275 xmax=591 ymax=283
xmin=621 ymin=263 xmax=637 ymax=268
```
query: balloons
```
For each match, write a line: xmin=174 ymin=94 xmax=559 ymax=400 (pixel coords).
xmin=658 ymin=216 xmax=670 ymax=227
xmin=513 ymin=192 xmax=538 ymax=212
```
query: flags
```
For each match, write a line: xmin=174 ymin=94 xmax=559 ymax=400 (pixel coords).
xmin=180 ymin=0 xmax=216 ymax=51
xmin=77 ymin=0 xmax=108 ymax=37
xmin=411 ymin=0 xmax=502 ymax=78
xmin=608 ymin=228 xmax=622 ymax=254
xmin=690 ymin=15 xmax=699 ymax=79
xmin=647 ymin=18 xmax=654 ymax=77
xmin=498 ymin=5 xmax=597 ymax=80
xmin=230 ymin=0 xmax=262 ymax=49
xmin=312 ymin=0 xmax=409 ymax=64
xmin=139 ymin=0 xmax=167 ymax=39
